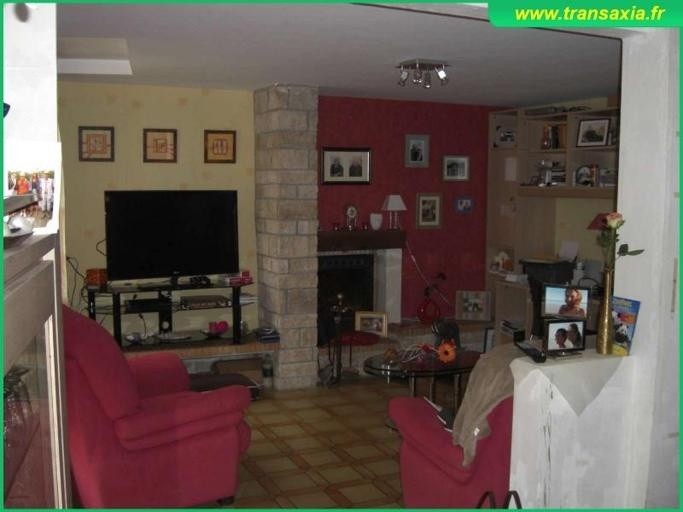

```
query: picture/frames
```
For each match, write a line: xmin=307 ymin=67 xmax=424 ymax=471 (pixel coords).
xmin=442 ymin=155 xmax=470 ymax=181
xmin=143 ymin=128 xmax=178 ymax=163
xmin=404 ymin=134 xmax=430 ymax=168
xmin=455 ymin=195 xmax=475 ymax=216
xmin=204 ymin=129 xmax=237 ymax=165
xmin=415 ymin=191 xmax=442 ymax=229
xmin=354 ymin=311 xmax=389 ymax=339
xmin=78 ymin=126 xmax=115 ymax=163
xmin=543 ymin=320 xmax=587 ymax=354
xmin=455 ymin=289 xmax=492 ymax=322
xmin=321 ymin=146 xmax=373 ymax=185
xmin=575 ymin=119 xmax=612 ymax=147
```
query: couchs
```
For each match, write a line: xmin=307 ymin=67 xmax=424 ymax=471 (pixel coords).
xmin=53 ymin=298 xmax=252 ymax=509
xmin=387 ymin=355 xmax=512 ymax=510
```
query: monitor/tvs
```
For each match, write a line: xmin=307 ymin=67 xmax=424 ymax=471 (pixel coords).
xmin=103 ymin=189 xmax=240 ymax=290
xmin=542 ymin=318 xmax=587 ymax=360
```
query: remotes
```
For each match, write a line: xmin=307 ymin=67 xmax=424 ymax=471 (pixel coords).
xmin=517 ymin=342 xmax=546 ymax=366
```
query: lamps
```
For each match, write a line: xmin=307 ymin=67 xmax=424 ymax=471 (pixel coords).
xmin=380 ymin=195 xmax=409 ymax=230
xmin=393 ymin=58 xmax=450 ymax=90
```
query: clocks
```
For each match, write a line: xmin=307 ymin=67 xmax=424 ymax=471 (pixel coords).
xmin=344 ymin=205 xmax=358 ymax=228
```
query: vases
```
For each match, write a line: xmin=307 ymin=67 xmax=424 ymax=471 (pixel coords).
xmin=596 ymin=272 xmax=615 ymax=357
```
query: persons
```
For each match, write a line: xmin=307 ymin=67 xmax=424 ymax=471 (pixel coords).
xmin=348 ymin=158 xmax=361 ymax=177
xmin=557 ymin=287 xmax=587 ymax=318
xmin=330 ymin=157 xmax=342 ymax=177
xmin=554 ymin=324 xmax=581 ymax=350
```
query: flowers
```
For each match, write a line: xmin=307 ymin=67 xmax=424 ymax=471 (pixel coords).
xmin=595 ymin=211 xmax=645 ymax=270
xmin=419 ymin=341 xmax=457 ymax=364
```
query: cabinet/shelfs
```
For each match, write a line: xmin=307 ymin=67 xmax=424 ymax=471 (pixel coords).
xmin=77 ymin=278 xmax=277 ymax=397
xmin=484 ymin=108 xmax=618 ymax=198
xmin=487 ymin=271 xmax=535 ymax=338
xmin=2 ymin=232 xmax=62 ymax=381
